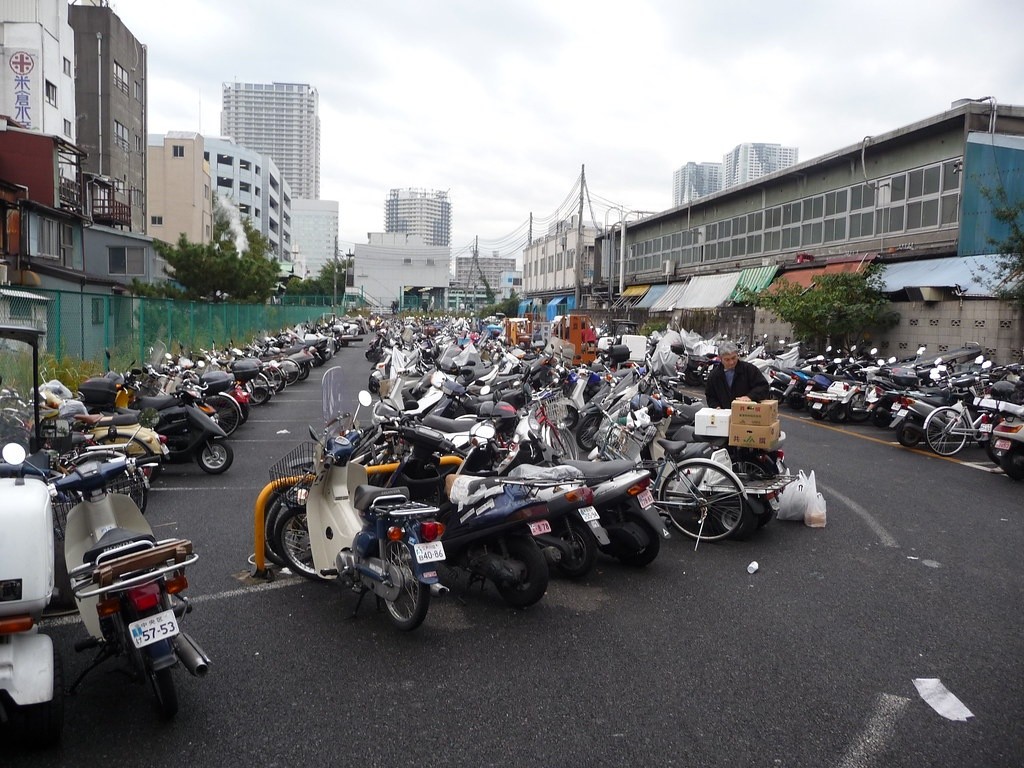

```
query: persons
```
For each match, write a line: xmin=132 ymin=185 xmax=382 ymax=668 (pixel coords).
xmin=459 ymin=301 xmax=474 ymax=312
xmin=452 ymin=316 xmax=470 ymax=330
xmin=391 ymin=300 xmax=434 ymax=315
xmin=368 ymin=312 xmax=375 ymax=325
xmin=705 ymin=341 xmax=770 ymax=409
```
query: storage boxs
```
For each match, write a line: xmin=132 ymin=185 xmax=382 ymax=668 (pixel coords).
xmin=728 ymin=420 xmax=781 ymax=448
xmin=731 ymin=399 xmax=778 ymax=426
xmin=695 ymin=407 xmax=731 ymax=436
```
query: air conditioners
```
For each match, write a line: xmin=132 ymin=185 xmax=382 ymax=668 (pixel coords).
xmin=662 ymin=260 xmax=675 ymax=275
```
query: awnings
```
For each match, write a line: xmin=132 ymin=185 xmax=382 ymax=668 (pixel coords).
xmin=610 ymin=253 xmax=1024 ymax=313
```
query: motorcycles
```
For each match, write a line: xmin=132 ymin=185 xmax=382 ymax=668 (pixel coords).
xmin=1 ymin=304 xmax=1024 ymax=746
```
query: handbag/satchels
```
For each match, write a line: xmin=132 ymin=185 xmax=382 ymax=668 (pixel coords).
xmin=777 ymin=469 xmax=818 ymax=522
xmin=804 ymin=491 xmax=828 ymax=529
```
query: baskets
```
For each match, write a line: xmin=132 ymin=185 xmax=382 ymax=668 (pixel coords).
xmin=0 ymin=414 xmax=74 ymax=455
xmin=51 ymin=470 xmax=135 ymax=542
xmin=539 ymin=391 xmax=569 ymax=420
xmin=602 ymin=406 xmax=657 ymax=459
xmin=269 ymin=438 xmax=322 ymax=508
xmin=138 ymin=372 xmax=162 ymax=397
